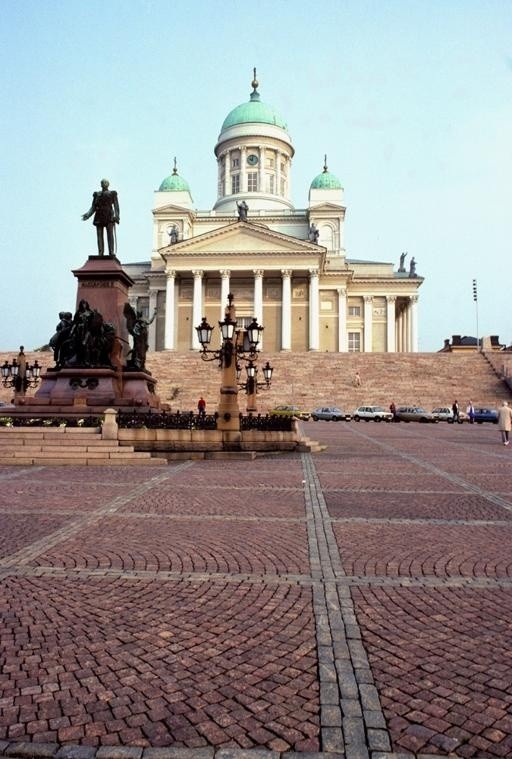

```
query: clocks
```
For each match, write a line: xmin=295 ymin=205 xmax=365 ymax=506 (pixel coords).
xmin=247 ymin=155 xmax=258 ymax=165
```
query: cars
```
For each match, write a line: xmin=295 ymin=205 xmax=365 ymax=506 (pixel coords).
xmin=394 ymin=406 xmax=433 ymax=423
xmin=474 ymin=407 xmax=498 ymax=424
xmin=310 ymin=406 xmax=353 ymax=422
xmin=352 ymin=405 xmax=394 ymax=422
xmin=431 ymin=406 xmax=471 ymax=424
xmin=268 ymin=403 xmax=312 ymax=421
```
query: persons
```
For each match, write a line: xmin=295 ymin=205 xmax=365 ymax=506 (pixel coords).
xmin=410 ymin=256 xmax=417 ymax=273
xmin=310 ymin=223 xmax=319 ymax=241
xmin=390 ymin=401 xmax=396 ymax=422
xmin=81 ymin=178 xmax=120 ymax=256
xmin=352 ymin=373 xmax=360 ymax=388
xmin=452 ymin=400 xmax=463 ymax=424
xmin=466 ymin=400 xmax=475 ymax=424
xmin=236 ymin=200 xmax=249 ymax=220
xmin=400 ymin=252 xmax=407 ymax=269
xmin=497 ymin=401 xmax=512 ymax=445
xmin=198 ymin=397 xmax=206 ymax=416
xmin=50 ymin=298 xmax=158 ymax=370
xmin=168 ymin=226 xmax=178 ymax=243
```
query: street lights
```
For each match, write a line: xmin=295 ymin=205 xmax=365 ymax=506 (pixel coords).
xmin=194 ymin=292 xmax=266 ymax=432
xmin=234 ymin=356 xmax=273 ymax=416
xmin=0 ymin=345 xmax=42 ymax=398
